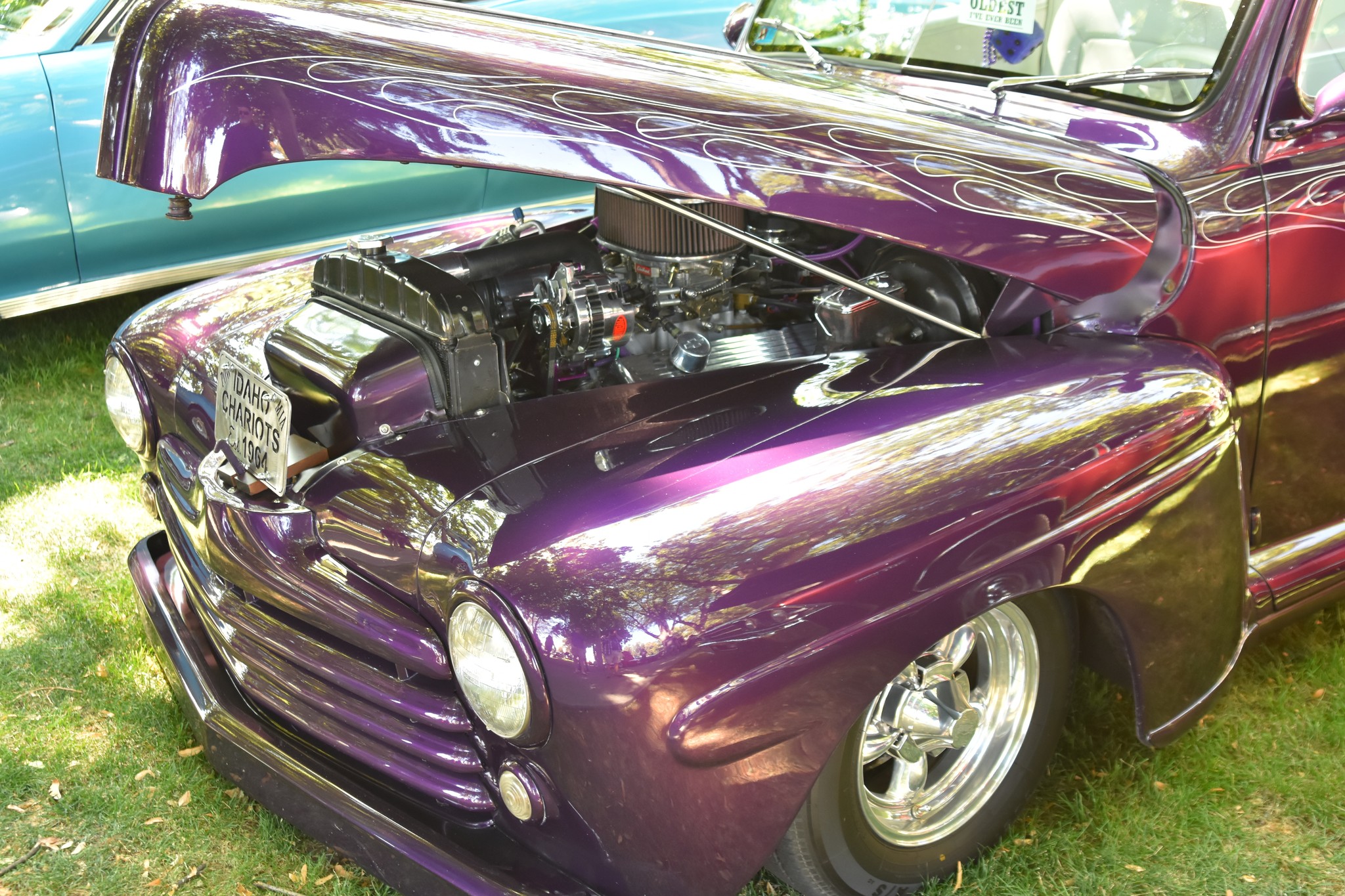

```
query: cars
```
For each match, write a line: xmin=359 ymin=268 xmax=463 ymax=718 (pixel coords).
xmin=1 ymin=0 xmax=750 ymax=332
xmin=95 ymin=0 xmax=1345 ymax=896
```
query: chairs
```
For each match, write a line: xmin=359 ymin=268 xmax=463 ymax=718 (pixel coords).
xmin=1040 ymin=0 xmax=1226 ymax=90
